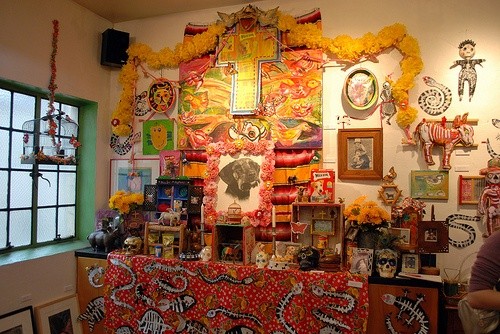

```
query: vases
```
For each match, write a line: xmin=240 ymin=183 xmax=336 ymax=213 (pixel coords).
xmin=363 ymin=226 xmax=376 ymax=248
xmin=125 ymin=211 xmax=145 ymax=241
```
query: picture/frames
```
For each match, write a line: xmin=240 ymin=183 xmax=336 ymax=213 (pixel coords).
xmin=401 ymin=253 xmax=418 ymax=273
xmin=418 ymin=221 xmax=449 ymax=254
xmin=391 ymin=228 xmax=411 ymax=245
xmin=338 ymin=128 xmax=383 ymax=180
xmin=109 ymin=158 xmax=161 ymax=201
xmin=411 ymin=169 xmax=449 ymax=200
xmin=0 ymin=305 xmax=36 ymax=334
xmin=34 ymin=294 xmax=83 ymax=334
xmin=458 ymin=174 xmax=485 ymax=204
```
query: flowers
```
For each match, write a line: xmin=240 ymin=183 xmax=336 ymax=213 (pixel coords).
xmin=108 ymin=192 xmax=144 ymax=214
xmin=343 ymin=197 xmax=391 ymax=227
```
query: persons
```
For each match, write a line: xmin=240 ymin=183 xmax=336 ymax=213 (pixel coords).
xmin=353 ymin=138 xmax=366 ymax=157
xmin=456 ymin=39 xmax=485 ymax=98
xmin=468 ymin=231 xmax=500 ymax=334
xmin=478 ymin=159 xmax=500 ymax=237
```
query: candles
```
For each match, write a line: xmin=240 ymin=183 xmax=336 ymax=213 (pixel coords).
xmin=201 ymin=203 xmax=205 ymax=224
xmin=272 ymin=205 xmax=276 ymax=227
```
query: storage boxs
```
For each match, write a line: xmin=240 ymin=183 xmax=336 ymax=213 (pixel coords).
xmin=309 ymin=169 xmax=336 ymax=204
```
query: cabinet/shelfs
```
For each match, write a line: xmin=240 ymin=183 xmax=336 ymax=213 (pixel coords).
xmin=211 ymin=222 xmax=256 ymax=266
xmin=290 ymin=203 xmax=345 ymax=271
xmin=145 ymin=178 xmax=200 ymax=260
xmin=74 ymin=248 xmax=442 ymax=334
